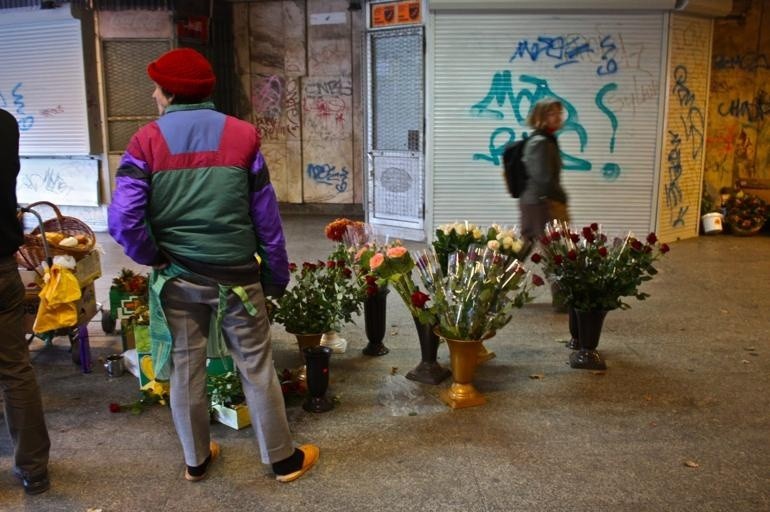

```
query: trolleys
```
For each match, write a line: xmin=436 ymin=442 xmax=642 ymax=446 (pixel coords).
xmin=13 ymin=207 xmax=117 ymax=366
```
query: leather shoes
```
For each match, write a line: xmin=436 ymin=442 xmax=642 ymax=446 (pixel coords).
xmin=18 ymin=476 xmax=50 ymax=494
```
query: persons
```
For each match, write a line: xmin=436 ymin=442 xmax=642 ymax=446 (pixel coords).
xmin=108 ymin=48 xmax=319 ymax=483
xmin=519 ymin=98 xmax=567 ymax=238
xmin=0 ymin=107 xmax=51 ymax=495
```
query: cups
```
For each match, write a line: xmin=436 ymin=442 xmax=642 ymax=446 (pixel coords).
xmin=104 ymin=355 xmax=125 ymax=377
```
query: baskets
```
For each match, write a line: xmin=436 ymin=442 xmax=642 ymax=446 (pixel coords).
xmin=14 ymin=201 xmax=96 ymax=272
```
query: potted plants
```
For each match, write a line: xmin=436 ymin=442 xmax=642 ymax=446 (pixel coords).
xmin=701 ymin=194 xmax=724 ymax=234
xmin=210 ymin=370 xmax=251 ymax=430
xmin=275 ymin=279 xmax=345 ymax=356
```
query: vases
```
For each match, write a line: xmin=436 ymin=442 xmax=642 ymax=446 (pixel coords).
xmin=406 ymin=320 xmax=452 ymax=386
xmin=436 ymin=326 xmax=487 ymax=410
xmin=567 ymin=304 xmax=609 ymax=370
xmin=473 ymin=326 xmax=496 ymax=364
xmin=320 ymin=329 xmax=348 ymax=355
xmin=303 ymin=346 xmax=333 ymax=412
xmin=361 ymin=289 xmax=391 ymax=355
xmin=730 ymin=224 xmax=762 ymax=237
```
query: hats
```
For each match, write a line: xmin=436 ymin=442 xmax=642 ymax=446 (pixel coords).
xmin=148 ymin=47 xmax=216 ymax=94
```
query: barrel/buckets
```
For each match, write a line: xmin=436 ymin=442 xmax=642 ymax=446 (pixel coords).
xmin=702 ymin=212 xmax=723 ymax=233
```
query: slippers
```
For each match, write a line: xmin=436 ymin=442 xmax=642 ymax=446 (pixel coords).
xmin=185 ymin=440 xmax=222 ymax=482
xmin=277 ymin=443 xmax=321 ymax=481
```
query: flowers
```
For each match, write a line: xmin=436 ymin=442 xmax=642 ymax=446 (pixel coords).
xmin=531 ymin=220 xmax=671 ymax=308
xmin=355 ymin=238 xmax=438 ymax=327
xmin=431 ymin=220 xmax=529 ymax=260
xmin=416 ymin=243 xmax=530 ymax=330
xmin=290 ymin=258 xmax=349 ymax=355
xmin=728 ymin=193 xmax=765 ymax=226
xmin=325 ymin=220 xmax=385 ymax=288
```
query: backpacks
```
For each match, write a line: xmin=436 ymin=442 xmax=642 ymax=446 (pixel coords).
xmin=502 ymin=131 xmax=550 ymax=198
